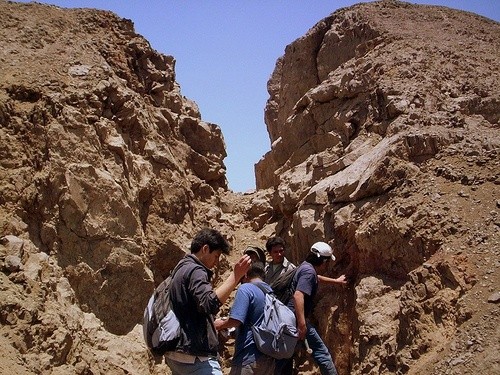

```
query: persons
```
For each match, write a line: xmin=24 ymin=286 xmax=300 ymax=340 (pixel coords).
xmin=143 ymin=228 xmax=349 ymax=375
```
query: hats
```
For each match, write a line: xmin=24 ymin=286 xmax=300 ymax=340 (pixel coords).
xmin=310 ymin=242 xmax=336 ymax=261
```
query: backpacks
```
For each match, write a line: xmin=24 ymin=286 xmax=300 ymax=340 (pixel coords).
xmin=143 ymin=260 xmax=197 ymax=356
xmin=246 ymin=282 xmax=298 ymax=359
xmin=270 ymin=261 xmax=313 ymax=306
